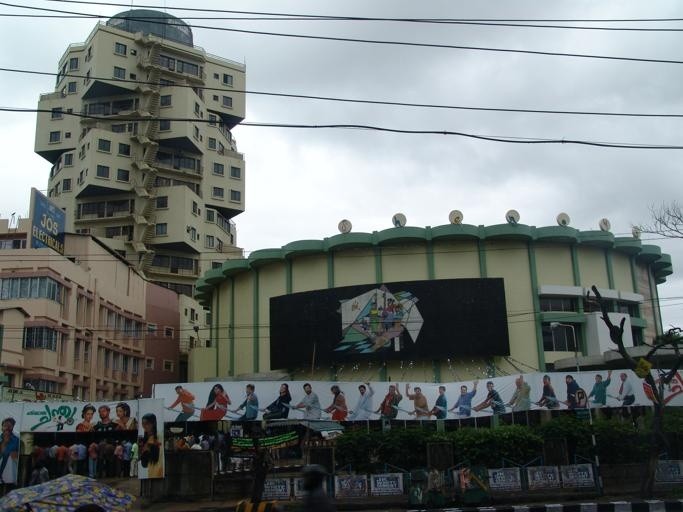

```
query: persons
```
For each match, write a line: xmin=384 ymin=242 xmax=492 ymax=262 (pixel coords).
xmin=92 ymin=405 xmax=117 ymax=432
xmin=298 ymin=464 xmax=330 ymax=511
xmin=138 ymin=413 xmax=160 ymax=477
xmin=0 ymin=417 xmax=23 ymax=497
xmin=166 ymin=370 xmax=637 ymax=423
xmin=30 ymin=429 xmax=234 ymax=484
xmin=76 ymin=404 xmax=95 ymax=433
xmin=112 ymin=402 xmax=138 ymax=431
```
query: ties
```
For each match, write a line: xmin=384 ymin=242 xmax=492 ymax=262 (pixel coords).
xmin=618 ymin=382 xmax=624 ymax=395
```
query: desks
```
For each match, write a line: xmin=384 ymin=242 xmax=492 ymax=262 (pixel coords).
xmin=139 ymin=448 xmax=215 ymax=501
